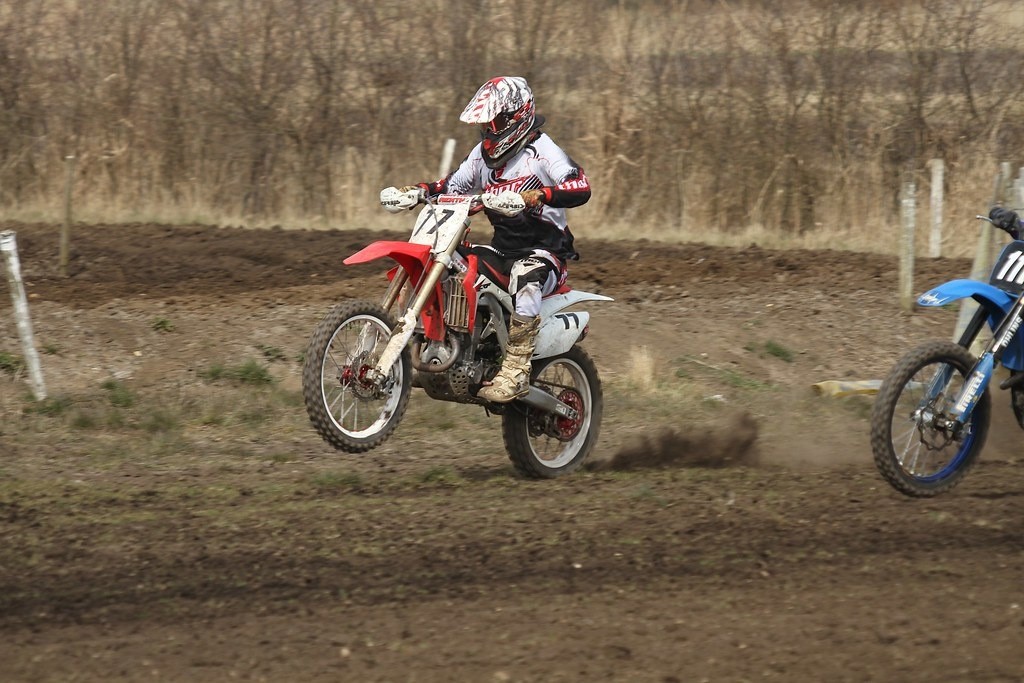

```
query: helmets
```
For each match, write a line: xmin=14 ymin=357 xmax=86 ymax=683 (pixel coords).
xmin=460 ymin=76 xmax=535 ymax=160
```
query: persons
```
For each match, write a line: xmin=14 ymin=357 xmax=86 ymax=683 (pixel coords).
xmin=380 ymin=76 xmax=592 ymax=404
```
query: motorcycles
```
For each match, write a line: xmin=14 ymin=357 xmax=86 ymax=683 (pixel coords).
xmin=301 ymin=187 xmax=615 ymax=482
xmin=870 ymin=216 xmax=1024 ymax=498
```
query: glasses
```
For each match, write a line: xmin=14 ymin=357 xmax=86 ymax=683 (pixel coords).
xmin=482 ymin=115 xmax=510 ymax=135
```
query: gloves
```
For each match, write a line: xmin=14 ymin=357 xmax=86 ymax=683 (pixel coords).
xmin=520 ymin=190 xmax=544 ymax=215
xmin=989 ymin=206 xmax=1023 ymax=240
xmin=398 ymin=186 xmax=422 ymax=209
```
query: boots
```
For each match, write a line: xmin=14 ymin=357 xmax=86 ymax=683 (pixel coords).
xmin=477 ymin=311 xmax=540 ymax=402
